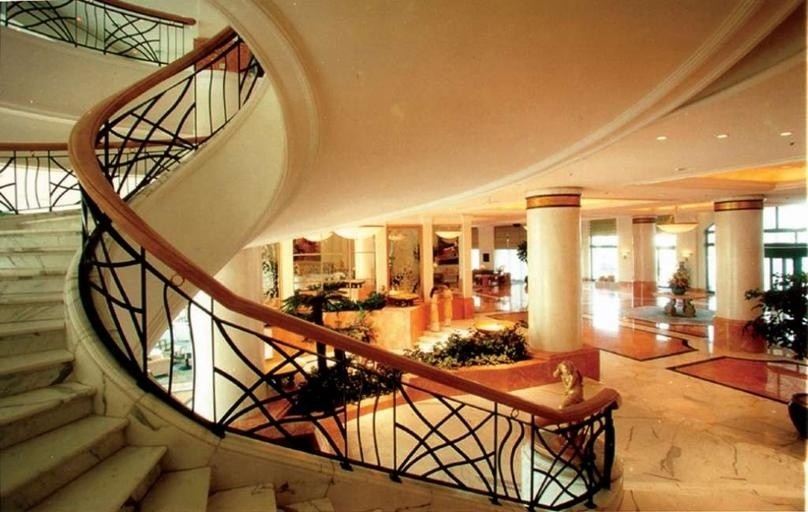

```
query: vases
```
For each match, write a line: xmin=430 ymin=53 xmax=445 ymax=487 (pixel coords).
xmin=671 ymin=286 xmax=686 ymax=295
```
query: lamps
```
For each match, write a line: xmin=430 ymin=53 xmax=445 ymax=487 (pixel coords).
xmin=303 ymin=232 xmax=334 ymax=242
xmin=679 ymin=251 xmax=693 ymax=260
xmin=621 ymin=249 xmax=631 ymax=258
xmin=335 ymin=222 xmax=385 ymax=241
xmin=653 ymin=204 xmax=700 ymax=235
xmin=432 ymin=206 xmax=465 ymax=241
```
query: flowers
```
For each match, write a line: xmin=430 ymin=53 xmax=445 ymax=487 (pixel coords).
xmin=668 ymin=270 xmax=691 ymax=289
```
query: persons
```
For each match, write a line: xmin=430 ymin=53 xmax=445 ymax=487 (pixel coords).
xmin=442 ymin=283 xmax=453 ymax=326
xmin=430 ymin=287 xmax=440 ymax=332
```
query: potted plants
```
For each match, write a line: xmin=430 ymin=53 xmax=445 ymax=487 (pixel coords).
xmin=739 ymin=270 xmax=808 ymax=442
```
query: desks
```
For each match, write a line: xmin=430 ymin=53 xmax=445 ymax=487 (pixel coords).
xmin=649 ymin=289 xmax=717 ymax=318
xmin=473 ymin=267 xmax=512 ymax=287
